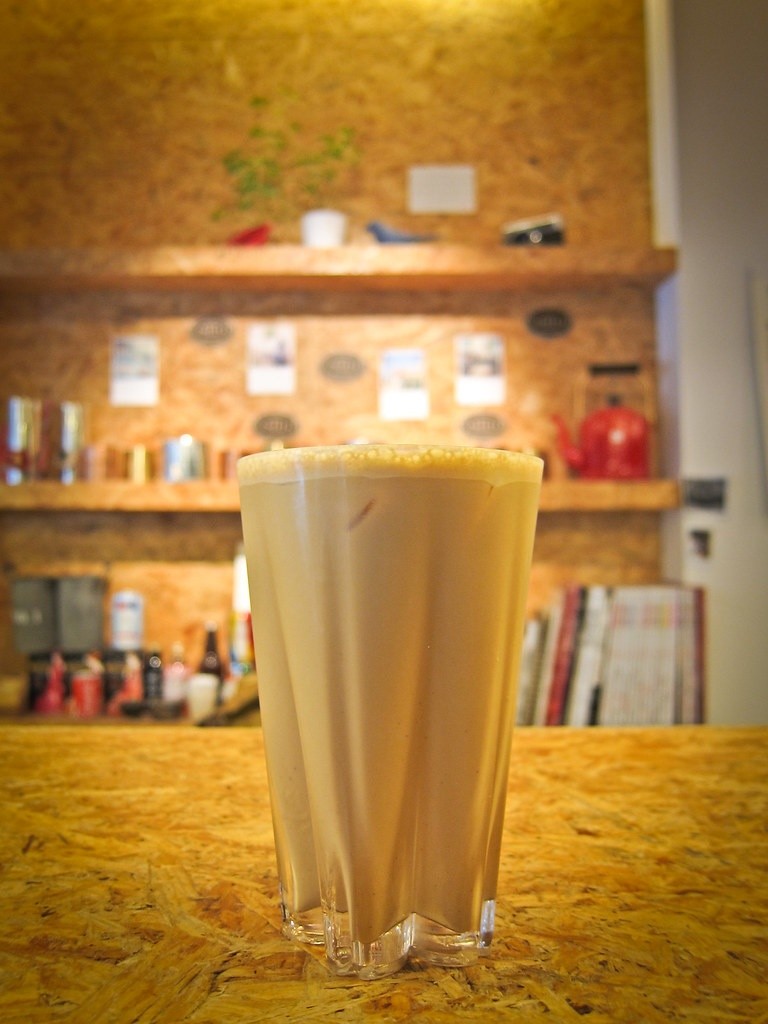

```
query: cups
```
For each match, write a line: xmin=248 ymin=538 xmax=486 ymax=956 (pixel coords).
xmin=302 ymin=209 xmax=343 ymax=247
xmin=191 ymin=674 xmax=218 ymax=721
xmin=237 ymin=444 xmax=544 ymax=979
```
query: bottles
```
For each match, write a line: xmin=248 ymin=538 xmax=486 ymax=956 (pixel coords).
xmin=189 ymin=620 xmax=226 ymax=705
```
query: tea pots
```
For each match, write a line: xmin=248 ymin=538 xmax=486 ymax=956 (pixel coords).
xmin=551 ymin=393 xmax=652 ymax=483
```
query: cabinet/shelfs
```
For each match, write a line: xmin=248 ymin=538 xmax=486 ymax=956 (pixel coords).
xmin=0 ymin=245 xmax=684 ymax=517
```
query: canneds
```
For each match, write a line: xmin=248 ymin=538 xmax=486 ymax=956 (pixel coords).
xmin=73 ymin=669 xmax=103 ymax=717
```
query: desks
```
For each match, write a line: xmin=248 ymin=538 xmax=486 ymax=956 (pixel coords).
xmin=0 ymin=725 xmax=768 ymax=1024
